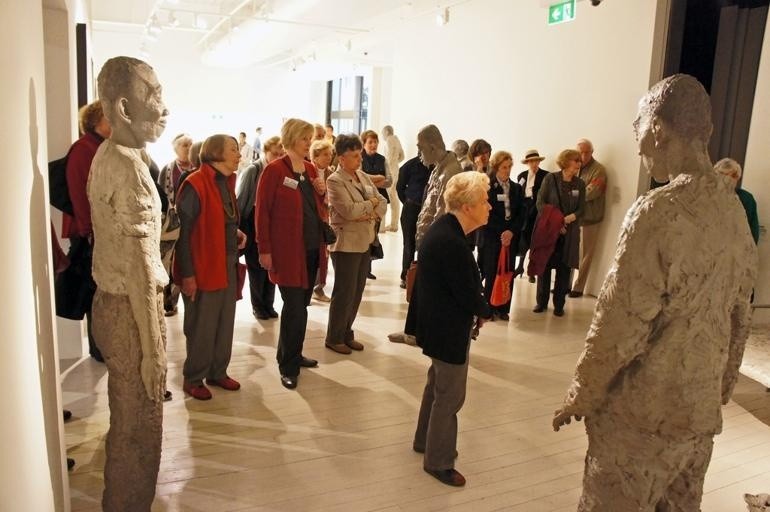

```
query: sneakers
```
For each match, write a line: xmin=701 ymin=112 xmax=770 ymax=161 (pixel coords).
xmin=183 ymin=382 xmax=212 ymax=399
xmin=206 ymin=377 xmax=240 ymax=390
xmin=164 ymin=306 xmax=178 ymax=316
xmin=401 ymin=280 xmax=405 ymax=288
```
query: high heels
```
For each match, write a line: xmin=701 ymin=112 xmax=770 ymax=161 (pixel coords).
xmin=529 ymin=276 xmax=535 ymax=283
xmin=514 ymin=269 xmax=524 ymax=277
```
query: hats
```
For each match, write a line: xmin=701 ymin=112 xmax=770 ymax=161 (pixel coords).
xmin=520 ymin=148 xmax=546 ymax=164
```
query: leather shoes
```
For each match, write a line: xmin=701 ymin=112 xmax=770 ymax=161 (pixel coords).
xmin=299 ymin=356 xmax=318 ymax=367
xmin=424 ymin=466 xmax=465 ymax=486
xmin=367 ymin=272 xmax=376 ymax=279
xmin=325 ymin=343 xmax=352 ymax=354
xmin=345 ymin=341 xmax=364 ymax=351
xmin=497 ymin=310 xmax=509 ymax=319
xmin=252 ymin=308 xmax=278 ymax=320
xmin=534 ymin=289 xmax=582 ymax=316
xmin=313 ymin=293 xmax=330 ymax=302
xmin=281 ymin=374 xmax=297 ymax=388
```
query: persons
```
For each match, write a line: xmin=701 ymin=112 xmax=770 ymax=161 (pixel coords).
xmin=395 ymin=138 xmax=548 ymax=320
xmin=159 ymin=117 xmax=404 ymax=400
xmin=714 ymin=158 xmax=759 ymax=247
xmin=61 ymin=99 xmax=111 ymax=363
xmin=552 ymin=73 xmax=760 ymax=511
xmin=412 ymin=170 xmax=492 ymax=486
xmin=387 ymin=123 xmax=461 ymax=346
xmin=536 ymin=138 xmax=607 ymax=317
xmin=88 ymin=56 xmax=170 ymax=512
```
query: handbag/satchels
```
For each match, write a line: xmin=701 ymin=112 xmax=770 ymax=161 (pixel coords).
xmin=49 ymin=156 xmax=73 ymax=216
xmin=235 ymin=261 xmax=248 ymax=300
xmin=490 ymin=272 xmax=513 ymax=307
xmin=321 ymin=221 xmax=336 ymax=245
xmin=55 ymin=235 xmax=93 ymax=319
xmin=369 ymin=243 xmax=383 ymax=260
xmin=407 ymin=261 xmax=418 ymax=304
